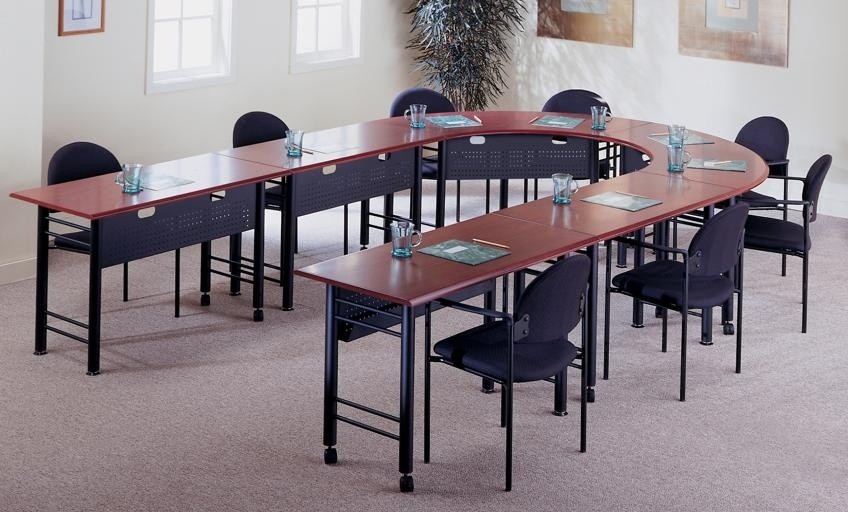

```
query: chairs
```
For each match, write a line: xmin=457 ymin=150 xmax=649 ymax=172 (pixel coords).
xmin=390 ymin=87 xmax=461 ymax=228
xmin=534 ymin=89 xmax=612 ymax=200
xmin=736 ymin=154 xmax=832 ymax=332
xmin=714 ymin=116 xmax=790 ymax=276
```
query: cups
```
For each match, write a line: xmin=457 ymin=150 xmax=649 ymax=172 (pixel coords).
xmin=667 ymin=144 xmax=692 ymax=172
xmin=390 ymin=222 xmax=422 ymax=258
xmin=283 ymin=129 xmax=305 ymax=157
xmin=115 ymin=162 xmax=144 ymax=194
xmin=667 ymin=123 xmax=689 ymax=146
xmin=552 ymin=172 xmax=579 ymax=205
xmin=590 ymin=105 xmax=613 ymax=130
xmin=405 ymin=103 xmax=426 ymax=127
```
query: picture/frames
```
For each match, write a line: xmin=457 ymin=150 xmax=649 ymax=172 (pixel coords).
xmin=58 ymin=0 xmax=105 ymax=36
xmin=678 ymin=0 xmax=789 ymax=69
xmin=537 ymin=0 xmax=634 ymax=48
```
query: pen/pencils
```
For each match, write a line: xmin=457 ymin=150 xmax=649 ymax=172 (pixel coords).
xmin=616 ymin=191 xmax=646 ymax=199
xmin=530 ymin=116 xmax=540 ymax=123
xmin=651 ymin=133 xmax=676 ymax=136
xmin=473 ymin=115 xmax=482 ymax=124
xmin=115 ymin=180 xmax=143 ymax=191
xmin=472 ymin=238 xmax=510 ymax=249
xmin=291 ymin=144 xmax=313 ymax=154
xmin=705 ymin=161 xmax=733 ymax=166
